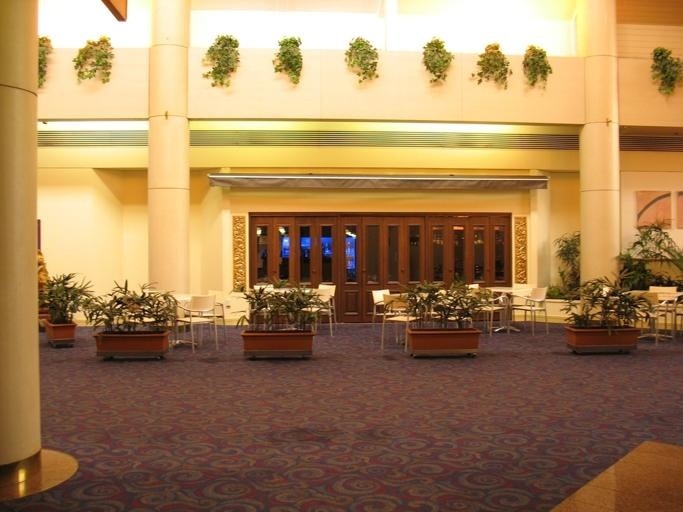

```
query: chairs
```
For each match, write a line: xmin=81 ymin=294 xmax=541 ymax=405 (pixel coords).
xmin=620 ymin=285 xmax=683 ymax=345
xmin=166 ymin=290 xmax=226 ymax=354
xmin=254 ymin=283 xmax=337 ymax=337
xmin=371 ymin=284 xmax=549 ymax=355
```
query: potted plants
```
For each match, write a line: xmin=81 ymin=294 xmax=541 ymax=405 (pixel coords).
xmin=38 ymin=273 xmax=95 ymax=347
xmin=228 ymin=279 xmax=335 ymax=360
xmin=82 ymin=279 xmax=180 ymax=362
xmin=384 ymin=277 xmax=493 ymax=357
xmin=559 ymin=276 xmax=654 ymax=354
